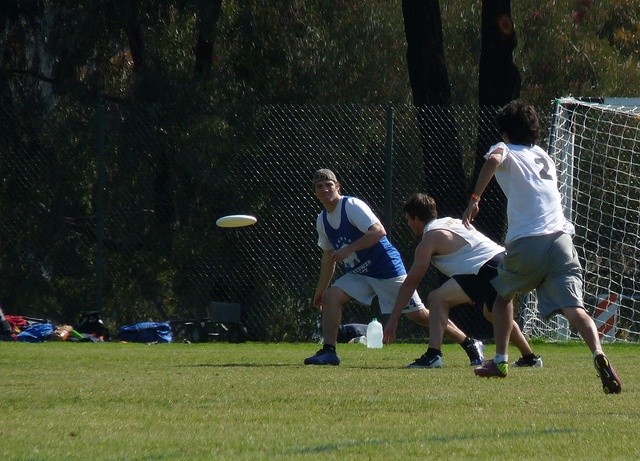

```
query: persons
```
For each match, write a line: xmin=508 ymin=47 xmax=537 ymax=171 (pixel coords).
xmin=382 ymin=193 xmax=544 ymax=370
xmin=304 ymin=168 xmax=485 ymax=367
xmin=463 ymin=99 xmax=622 ymax=396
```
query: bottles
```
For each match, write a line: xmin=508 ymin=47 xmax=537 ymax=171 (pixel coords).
xmin=366 ymin=318 xmax=384 ymax=349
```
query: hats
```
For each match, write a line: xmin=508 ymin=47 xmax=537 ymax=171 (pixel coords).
xmin=312 ymin=169 xmax=337 ymax=183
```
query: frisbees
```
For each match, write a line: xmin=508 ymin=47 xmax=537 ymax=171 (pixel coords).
xmin=216 ymin=215 xmax=257 ymax=228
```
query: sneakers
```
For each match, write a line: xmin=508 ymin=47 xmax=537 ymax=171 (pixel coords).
xmin=463 ymin=339 xmax=484 ymax=365
xmin=304 ymin=348 xmax=340 ymax=365
xmin=474 ymin=360 xmax=509 ymax=378
xmin=592 ymin=351 xmax=621 ymax=394
xmin=511 ymin=357 xmax=543 ymax=368
xmin=406 ymin=352 xmax=443 ymax=368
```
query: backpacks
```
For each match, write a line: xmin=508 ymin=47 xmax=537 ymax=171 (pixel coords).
xmin=121 ymin=322 xmax=173 ymax=343
xmin=337 ymin=323 xmax=368 ymax=343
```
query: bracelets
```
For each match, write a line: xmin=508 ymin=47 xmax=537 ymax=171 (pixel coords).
xmin=471 ymin=194 xmax=481 ymax=202
